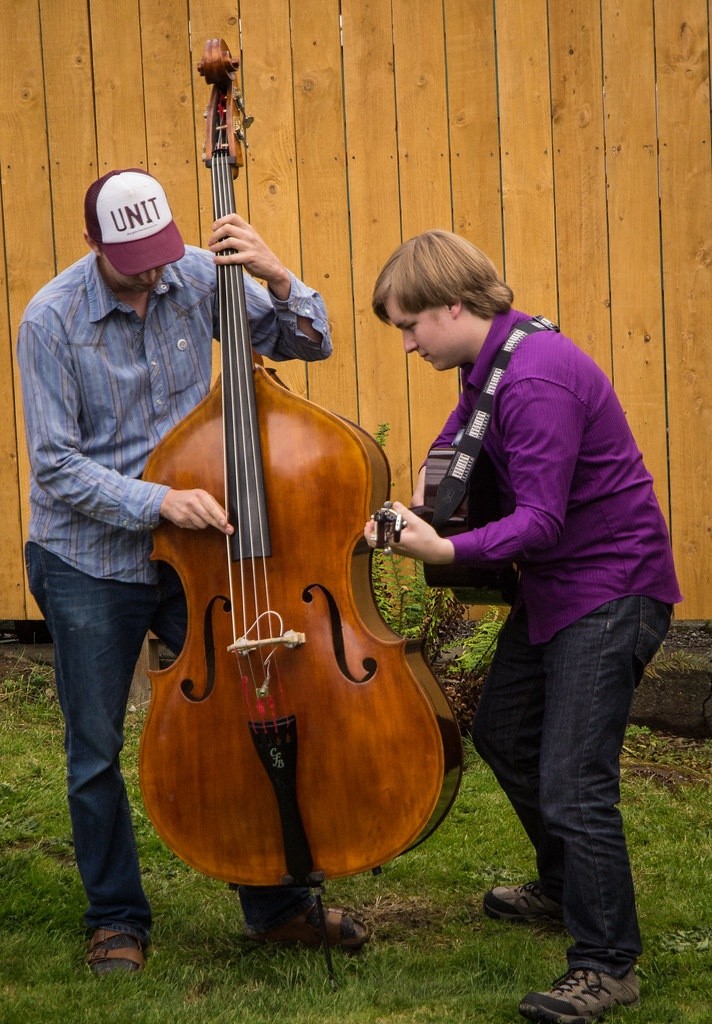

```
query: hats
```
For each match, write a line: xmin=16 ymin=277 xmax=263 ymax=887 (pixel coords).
xmin=84 ymin=168 xmax=186 ymax=276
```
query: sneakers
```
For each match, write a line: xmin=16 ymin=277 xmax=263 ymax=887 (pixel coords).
xmin=483 ymin=882 xmax=566 ymax=920
xmin=520 ymin=965 xmax=642 ymax=1024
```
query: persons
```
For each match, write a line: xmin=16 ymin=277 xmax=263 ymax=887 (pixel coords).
xmin=363 ymin=229 xmax=682 ymax=1024
xmin=17 ymin=166 xmax=371 ymax=978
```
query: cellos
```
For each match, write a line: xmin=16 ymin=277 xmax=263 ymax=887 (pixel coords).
xmin=137 ymin=34 xmax=464 ymax=978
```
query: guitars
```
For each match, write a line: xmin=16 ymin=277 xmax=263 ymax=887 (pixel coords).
xmin=363 ymin=439 xmax=513 ymax=606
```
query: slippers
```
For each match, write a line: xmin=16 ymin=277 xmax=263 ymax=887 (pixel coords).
xmin=242 ymin=901 xmax=372 ymax=946
xmin=86 ymin=924 xmax=145 ymax=981
